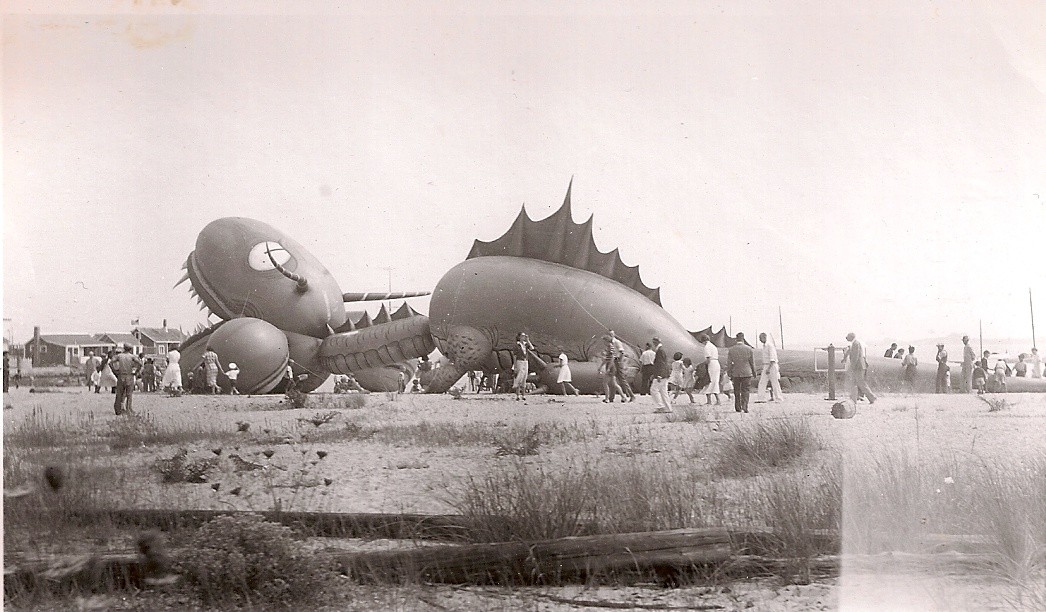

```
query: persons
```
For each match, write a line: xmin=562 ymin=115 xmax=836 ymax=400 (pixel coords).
xmin=409 ymin=379 xmax=419 ymax=393
xmin=398 ymin=365 xmax=407 ymax=394
xmin=434 ymin=352 xmax=449 ymax=394
xmin=511 ymin=331 xmax=536 ymax=401
xmin=553 ymin=346 xmax=580 ymax=397
xmin=80 ymin=342 xmax=204 ymax=416
xmin=190 ymin=344 xmax=225 ymax=395
xmin=3 ymin=351 xmax=11 ymax=392
xmin=464 ymin=367 xmax=497 ymax=393
xmin=284 ymin=359 xmax=297 ymax=395
xmin=223 ymin=362 xmax=240 ymax=395
xmin=583 ymin=319 xmax=1046 ymax=422
xmin=416 ymin=355 xmax=433 ymax=389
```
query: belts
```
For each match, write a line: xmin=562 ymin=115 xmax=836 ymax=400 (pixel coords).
xmin=614 ymin=358 xmax=620 ymax=360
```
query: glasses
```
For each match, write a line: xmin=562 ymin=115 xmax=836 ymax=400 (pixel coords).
xmin=520 ymin=335 xmax=526 ymax=337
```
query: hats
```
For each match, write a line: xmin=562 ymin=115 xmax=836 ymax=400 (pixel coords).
xmin=1018 ymin=354 xmax=1024 ymax=357
xmin=228 ymin=363 xmax=237 ymax=370
xmin=287 ymin=359 xmax=294 ymax=362
xmin=936 ymin=341 xmax=944 ymax=347
xmin=962 ymin=336 xmax=969 ymax=341
xmin=88 ymin=351 xmax=94 ymax=355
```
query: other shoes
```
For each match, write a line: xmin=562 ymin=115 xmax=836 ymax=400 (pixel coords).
xmin=870 ymin=398 xmax=877 ymax=405
xmin=620 ymin=401 xmax=626 ymax=404
xmin=628 ymin=396 xmax=636 ymax=403
xmin=216 ymin=387 xmax=222 ymax=394
xmin=601 ymin=400 xmax=609 ymax=404
xmin=516 ymin=398 xmax=526 ymax=401
xmin=575 ymin=388 xmax=580 ymax=397
xmin=978 ymin=390 xmax=985 ymax=394
xmin=755 ymin=400 xmax=767 ymax=403
xmin=857 ymin=398 xmax=864 ymax=401
xmin=736 ymin=409 xmax=750 ymax=413
xmin=610 ymin=400 xmax=614 ymax=402
